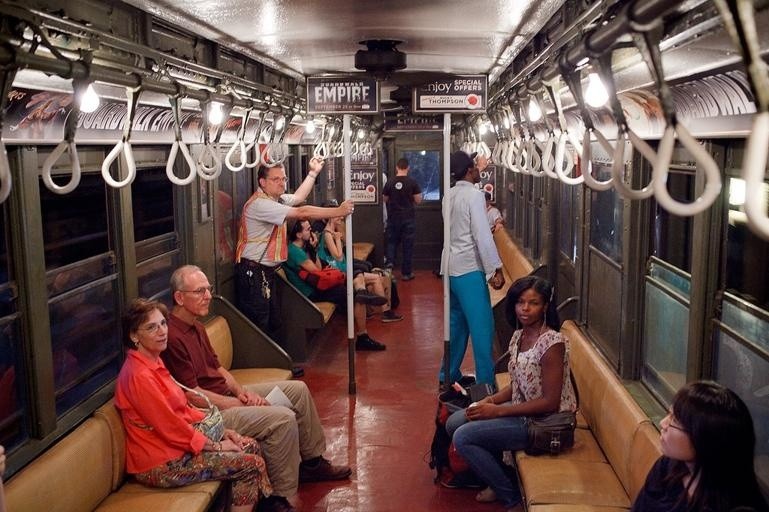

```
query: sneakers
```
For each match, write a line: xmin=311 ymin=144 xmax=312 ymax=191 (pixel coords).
xmin=354 ymin=289 xmax=388 ymax=306
xmin=366 ymin=311 xmax=375 ymax=319
xmin=356 ymin=336 xmax=386 ymax=352
xmin=403 ymin=273 xmax=415 ymax=280
xmin=382 ymin=312 xmax=405 ymax=322
xmin=385 ymin=264 xmax=394 ymax=269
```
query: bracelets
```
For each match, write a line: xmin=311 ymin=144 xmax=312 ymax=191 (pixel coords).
xmin=308 ymin=174 xmax=316 ymax=178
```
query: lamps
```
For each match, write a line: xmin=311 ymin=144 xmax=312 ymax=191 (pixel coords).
xmin=306 ymin=116 xmax=315 ymax=133
xmin=272 ymin=117 xmax=283 ymax=130
xmin=79 ymin=79 xmax=101 ymax=114
xmin=528 ymin=99 xmax=541 ymax=121
xmin=585 ymin=64 xmax=610 ymax=108
xmin=209 ymin=102 xmax=223 ymax=125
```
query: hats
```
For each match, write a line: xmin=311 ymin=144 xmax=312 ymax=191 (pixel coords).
xmin=450 ymin=150 xmax=477 ymax=176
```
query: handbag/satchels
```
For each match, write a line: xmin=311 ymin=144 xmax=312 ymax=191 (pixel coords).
xmin=515 ymin=328 xmax=581 ymax=457
xmin=285 ymin=261 xmax=346 ymax=292
xmin=129 ymin=374 xmax=225 ymax=444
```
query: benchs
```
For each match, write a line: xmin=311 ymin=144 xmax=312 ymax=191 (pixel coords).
xmin=486 ymin=221 xmax=534 ymax=309
xmin=277 ymin=242 xmax=374 ymax=321
xmin=493 ymin=320 xmax=677 ymax=511
xmin=0 ymin=316 xmax=292 ymax=511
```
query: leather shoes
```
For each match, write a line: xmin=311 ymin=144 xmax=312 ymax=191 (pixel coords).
xmin=293 ymin=366 xmax=303 ymax=377
xmin=475 ymin=487 xmax=497 ymax=502
xmin=439 ymin=375 xmax=475 ymax=388
xmin=254 ymin=494 xmax=293 ymax=512
xmin=298 ymin=456 xmax=353 ymax=484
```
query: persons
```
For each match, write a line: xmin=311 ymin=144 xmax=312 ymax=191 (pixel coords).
xmin=113 ymin=296 xmax=272 ymax=512
xmin=315 ymin=198 xmax=400 ymax=307
xmin=235 ymin=157 xmax=354 ymax=376
xmin=285 ymin=220 xmax=386 ymax=351
xmin=633 ymin=380 xmax=769 ymax=512
xmin=317 ymin=205 xmax=404 ymax=323
xmin=384 ymin=156 xmax=422 ymax=280
xmin=440 ymin=150 xmax=505 ymax=394
xmin=444 ymin=276 xmax=578 ymax=505
xmin=382 ymin=172 xmax=388 ymax=264
xmin=162 ymin=265 xmax=352 ymax=512
xmin=482 ymin=190 xmax=505 ymax=233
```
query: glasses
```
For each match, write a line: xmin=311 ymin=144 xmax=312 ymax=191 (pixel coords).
xmin=265 ymin=177 xmax=288 ymax=183
xmin=181 ymin=285 xmax=216 ymax=294
xmin=136 ymin=319 xmax=167 ymax=333
xmin=667 ymin=403 xmax=687 ymax=433
